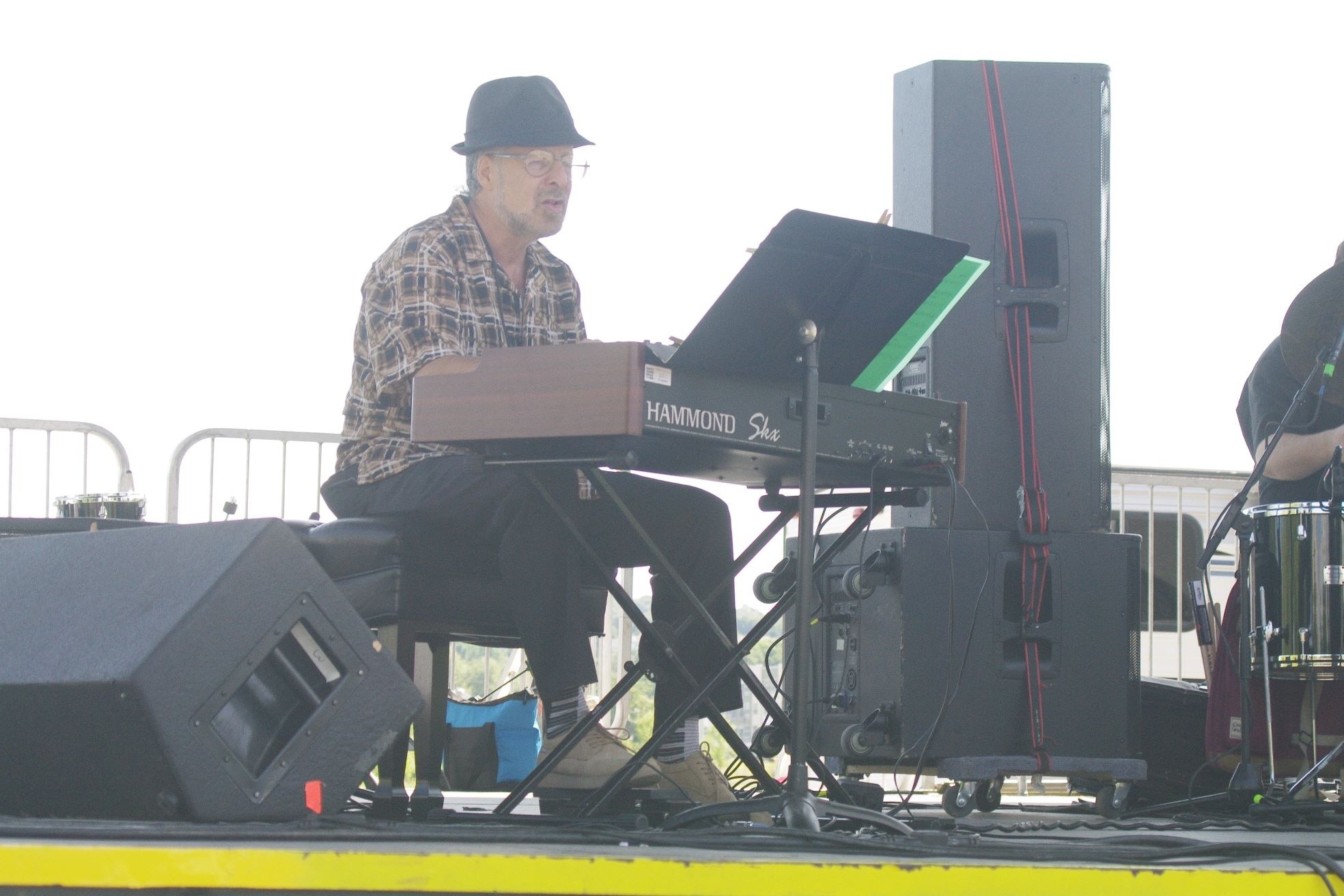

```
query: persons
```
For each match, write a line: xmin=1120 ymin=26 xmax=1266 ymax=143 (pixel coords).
xmin=332 ymin=75 xmax=746 ymax=805
xmin=1238 ymin=240 xmax=1344 ymax=650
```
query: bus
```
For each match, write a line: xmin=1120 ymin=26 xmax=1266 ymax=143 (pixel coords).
xmin=774 ymin=472 xmax=1263 ymax=789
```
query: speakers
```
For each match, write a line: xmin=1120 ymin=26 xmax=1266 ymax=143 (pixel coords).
xmin=891 ymin=61 xmax=1112 ymax=533
xmin=778 ymin=525 xmax=1142 ymax=765
xmin=0 ymin=519 xmax=425 ymax=826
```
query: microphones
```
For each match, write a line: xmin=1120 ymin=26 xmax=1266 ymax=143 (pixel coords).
xmin=1321 ymin=326 xmax=1344 ymax=387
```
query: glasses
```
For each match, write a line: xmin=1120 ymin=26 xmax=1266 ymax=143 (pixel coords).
xmin=492 ymin=149 xmax=588 ymax=180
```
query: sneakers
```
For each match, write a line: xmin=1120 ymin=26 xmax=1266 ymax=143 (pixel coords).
xmin=649 ymin=743 xmax=776 ymax=827
xmin=535 ymin=721 xmax=660 ymax=788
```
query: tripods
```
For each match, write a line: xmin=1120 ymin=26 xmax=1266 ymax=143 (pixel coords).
xmin=1118 ymin=345 xmax=1335 ymax=821
xmin=645 ymin=210 xmax=969 ymax=840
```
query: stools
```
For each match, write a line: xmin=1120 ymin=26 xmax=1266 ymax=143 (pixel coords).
xmin=286 ymin=517 xmax=617 ymax=820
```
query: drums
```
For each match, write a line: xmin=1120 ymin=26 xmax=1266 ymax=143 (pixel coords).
xmin=1237 ymin=501 xmax=1343 ymax=683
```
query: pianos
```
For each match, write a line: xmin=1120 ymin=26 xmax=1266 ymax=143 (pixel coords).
xmin=410 ymin=341 xmax=992 ymax=834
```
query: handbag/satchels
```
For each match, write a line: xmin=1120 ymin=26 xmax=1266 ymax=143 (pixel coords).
xmin=442 ymin=644 xmax=542 ymax=793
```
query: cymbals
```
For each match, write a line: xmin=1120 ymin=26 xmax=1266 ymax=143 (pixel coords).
xmin=1280 ymin=260 xmax=1344 ymax=408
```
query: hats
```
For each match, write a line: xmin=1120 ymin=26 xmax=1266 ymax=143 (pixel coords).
xmin=451 ymin=75 xmax=596 ymax=155
